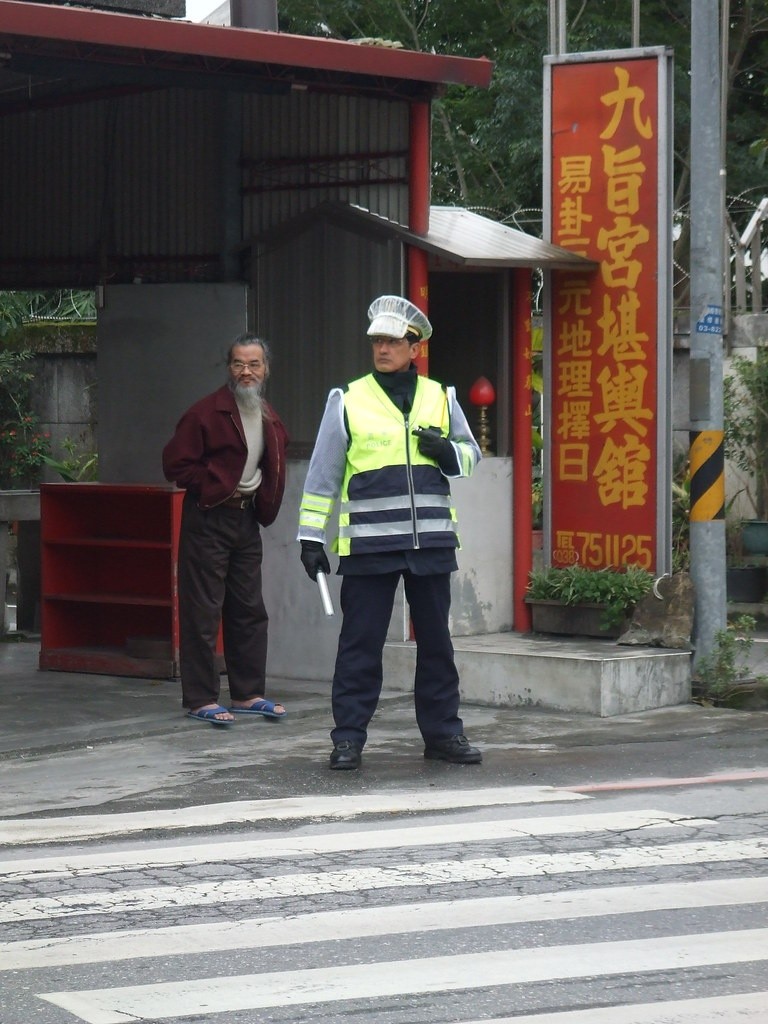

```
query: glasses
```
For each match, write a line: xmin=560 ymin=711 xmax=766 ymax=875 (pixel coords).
xmin=369 ymin=336 xmax=412 ymax=344
xmin=229 ymin=362 xmax=264 ymax=371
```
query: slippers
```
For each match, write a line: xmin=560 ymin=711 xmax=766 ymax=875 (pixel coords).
xmin=229 ymin=699 xmax=288 ymax=718
xmin=186 ymin=705 xmax=234 ymax=724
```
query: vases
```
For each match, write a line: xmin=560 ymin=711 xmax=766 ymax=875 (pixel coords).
xmin=522 ymin=565 xmax=658 ymax=638
xmin=726 ymin=562 xmax=768 ymax=603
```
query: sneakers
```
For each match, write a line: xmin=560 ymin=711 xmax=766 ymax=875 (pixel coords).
xmin=330 ymin=741 xmax=361 ymax=769
xmin=424 ymin=735 xmax=482 ymax=762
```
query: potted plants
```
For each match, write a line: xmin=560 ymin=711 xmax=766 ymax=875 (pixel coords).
xmin=532 ymin=480 xmax=543 ymax=550
xmin=722 ymin=337 xmax=768 ymax=553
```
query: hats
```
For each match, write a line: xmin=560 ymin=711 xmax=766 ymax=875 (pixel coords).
xmin=367 ymin=295 xmax=432 ymax=341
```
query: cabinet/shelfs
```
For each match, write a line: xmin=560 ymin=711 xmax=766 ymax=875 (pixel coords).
xmin=38 ymin=482 xmax=228 ymax=681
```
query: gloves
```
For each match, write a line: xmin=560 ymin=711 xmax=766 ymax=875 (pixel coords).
xmin=412 ymin=429 xmax=446 ymax=458
xmin=301 ymin=540 xmax=331 ymax=582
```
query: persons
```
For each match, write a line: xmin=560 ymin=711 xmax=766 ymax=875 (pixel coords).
xmin=161 ymin=333 xmax=285 ymax=718
xmin=296 ymin=295 xmax=482 ymax=771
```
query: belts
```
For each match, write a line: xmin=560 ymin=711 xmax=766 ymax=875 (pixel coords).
xmin=218 ymin=496 xmax=251 ymax=509
